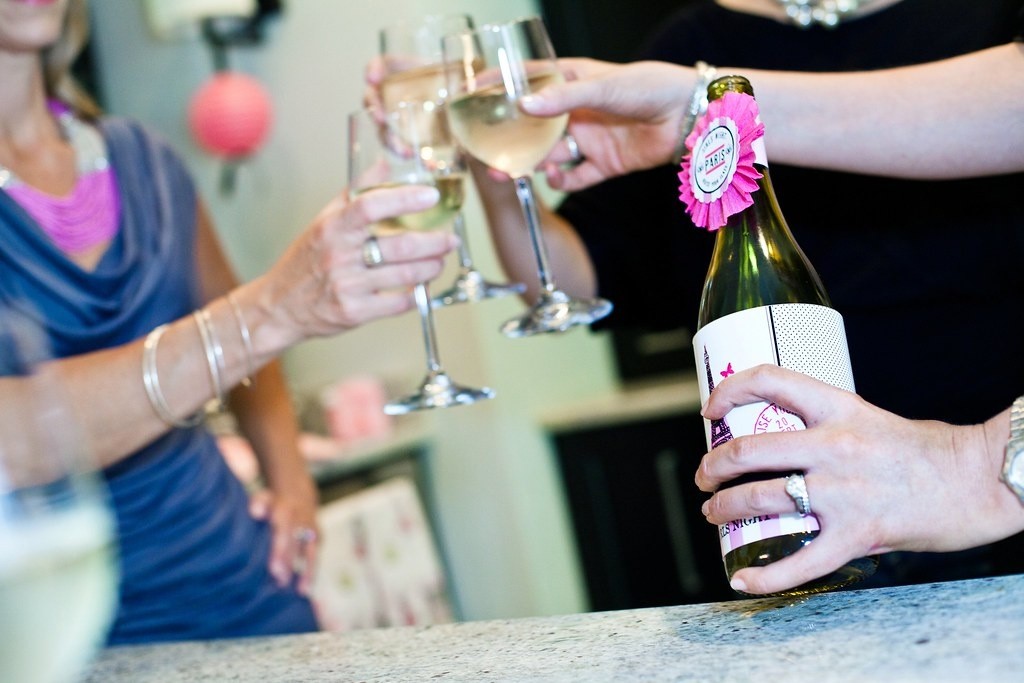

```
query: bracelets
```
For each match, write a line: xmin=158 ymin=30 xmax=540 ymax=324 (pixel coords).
xmin=221 ymin=287 xmax=258 ymax=374
xmin=192 ymin=305 xmax=229 ymax=407
xmin=141 ymin=323 xmax=207 ymax=427
xmin=672 ymin=61 xmax=716 ymax=165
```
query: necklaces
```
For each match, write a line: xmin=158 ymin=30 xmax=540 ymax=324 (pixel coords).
xmin=0 ymin=98 xmax=121 ymax=252
xmin=774 ymin=0 xmax=860 ymax=28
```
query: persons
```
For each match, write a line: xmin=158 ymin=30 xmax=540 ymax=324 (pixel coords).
xmin=463 ymin=43 xmax=1024 ymax=595
xmin=0 ymin=0 xmax=462 ymax=650
xmin=366 ymin=0 xmax=1024 ymax=585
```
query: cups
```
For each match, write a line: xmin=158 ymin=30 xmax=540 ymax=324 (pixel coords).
xmin=1 ymin=307 xmax=121 ymax=683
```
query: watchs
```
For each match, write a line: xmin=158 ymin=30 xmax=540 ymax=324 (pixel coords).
xmin=998 ymin=396 xmax=1024 ymax=506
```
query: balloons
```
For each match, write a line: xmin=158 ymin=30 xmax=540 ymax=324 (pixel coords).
xmin=194 ymin=72 xmax=271 ymax=156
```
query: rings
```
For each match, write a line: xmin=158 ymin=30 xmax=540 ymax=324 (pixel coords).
xmin=565 ymin=135 xmax=580 ymax=159
xmin=785 ymin=474 xmax=811 ymax=516
xmin=363 ymin=239 xmax=383 ymax=265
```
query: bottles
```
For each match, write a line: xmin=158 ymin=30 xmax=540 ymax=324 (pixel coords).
xmin=692 ymin=74 xmax=883 ymax=597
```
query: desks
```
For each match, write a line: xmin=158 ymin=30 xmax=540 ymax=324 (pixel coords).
xmin=80 ymin=575 xmax=1023 ymax=683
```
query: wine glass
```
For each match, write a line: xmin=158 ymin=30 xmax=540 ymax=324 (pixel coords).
xmin=348 ymin=12 xmax=612 ymax=415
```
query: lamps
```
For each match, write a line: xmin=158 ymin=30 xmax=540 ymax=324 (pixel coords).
xmin=143 ymin=0 xmax=281 ymax=48
